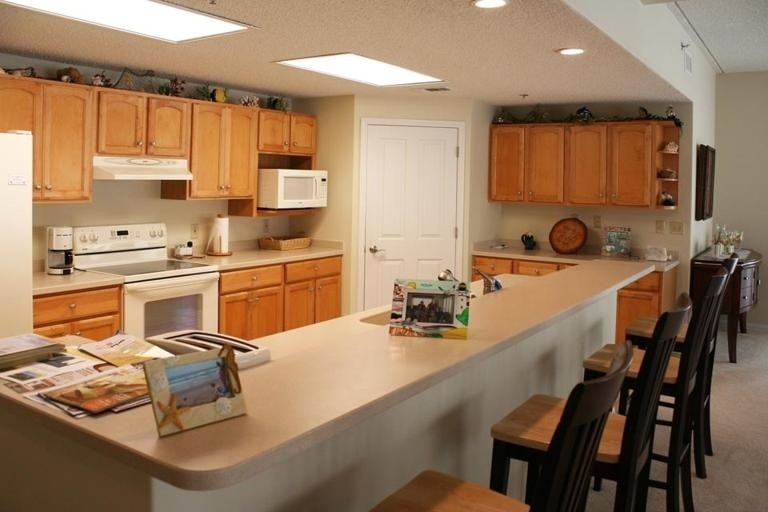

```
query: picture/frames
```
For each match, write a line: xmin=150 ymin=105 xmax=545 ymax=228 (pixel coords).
xmin=389 ymin=279 xmax=470 ymax=340
xmin=142 ymin=345 xmax=249 ymax=439
xmin=698 ymin=143 xmax=715 ymax=221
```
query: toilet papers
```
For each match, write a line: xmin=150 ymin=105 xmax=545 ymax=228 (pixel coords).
xmin=204 ymin=217 xmax=229 ymax=254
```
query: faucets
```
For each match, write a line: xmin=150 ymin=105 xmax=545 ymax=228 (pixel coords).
xmin=438 ymin=268 xmax=502 ymax=299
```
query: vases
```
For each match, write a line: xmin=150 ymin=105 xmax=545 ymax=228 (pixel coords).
xmin=714 ymin=243 xmax=724 ymax=258
xmin=726 ymin=244 xmax=735 ymax=255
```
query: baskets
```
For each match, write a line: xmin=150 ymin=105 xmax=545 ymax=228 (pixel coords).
xmin=260 ymin=231 xmax=311 ymax=251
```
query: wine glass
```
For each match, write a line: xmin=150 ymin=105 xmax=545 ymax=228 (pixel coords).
xmin=734 ymin=231 xmax=743 ymax=253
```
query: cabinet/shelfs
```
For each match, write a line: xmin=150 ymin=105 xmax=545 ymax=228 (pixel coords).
xmin=692 ymin=244 xmax=762 ymax=363
xmin=489 ymin=122 xmax=681 ymax=209
xmin=471 ymin=253 xmax=677 ymax=352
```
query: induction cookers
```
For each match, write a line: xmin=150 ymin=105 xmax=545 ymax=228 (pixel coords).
xmin=89 ymin=258 xmax=216 ymax=285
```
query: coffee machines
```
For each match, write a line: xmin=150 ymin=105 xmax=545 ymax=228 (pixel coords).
xmin=43 ymin=226 xmax=74 ymax=276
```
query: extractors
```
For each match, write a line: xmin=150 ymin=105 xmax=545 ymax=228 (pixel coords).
xmin=93 ymin=157 xmax=193 ymax=184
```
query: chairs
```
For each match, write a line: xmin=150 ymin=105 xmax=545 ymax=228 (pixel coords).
xmin=367 ymin=342 xmax=642 ymax=512
xmin=619 ymin=253 xmax=740 ymax=415
xmin=580 ymin=265 xmax=720 ymax=508
xmin=489 ymin=292 xmax=693 ymax=512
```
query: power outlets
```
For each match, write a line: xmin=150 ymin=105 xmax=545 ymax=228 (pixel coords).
xmin=191 ymin=223 xmax=199 ymax=239
xmin=262 ymin=219 xmax=270 ymax=234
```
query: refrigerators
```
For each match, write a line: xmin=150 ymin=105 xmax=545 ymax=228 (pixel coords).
xmin=0 ymin=130 xmax=34 ymax=337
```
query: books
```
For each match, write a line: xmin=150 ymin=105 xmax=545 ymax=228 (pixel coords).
xmin=0 ymin=332 xmax=177 ymax=419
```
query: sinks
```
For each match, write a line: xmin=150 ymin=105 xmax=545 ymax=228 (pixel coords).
xmin=359 ymin=310 xmax=391 ymax=326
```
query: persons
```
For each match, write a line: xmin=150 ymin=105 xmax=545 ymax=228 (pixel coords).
xmin=210 ymin=382 xmax=226 ymax=400
xmin=414 ymin=298 xmax=448 ymax=323
xmin=216 ymin=361 xmax=230 ymax=394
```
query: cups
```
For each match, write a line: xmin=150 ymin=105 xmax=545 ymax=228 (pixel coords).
xmin=522 ymin=231 xmax=536 ymax=251
xmin=713 ymin=245 xmax=722 ymax=258
xmin=724 ymin=245 xmax=732 ymax=256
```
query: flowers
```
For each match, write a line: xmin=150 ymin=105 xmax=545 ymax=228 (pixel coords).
xmin=726 ymin=231 xmax=745 ymax=246
xmin=160 ymin=76 xmax=186 ymax=96
xmin=712 ymin=224 xmax=728 ymax=244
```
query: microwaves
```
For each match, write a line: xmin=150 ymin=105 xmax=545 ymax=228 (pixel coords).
xmin=259 ymin=167 xmax=329 ymax=210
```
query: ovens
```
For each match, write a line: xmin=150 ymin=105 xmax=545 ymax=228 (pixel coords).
xmin=122 ymin=272 xmax=220 ymax=337
